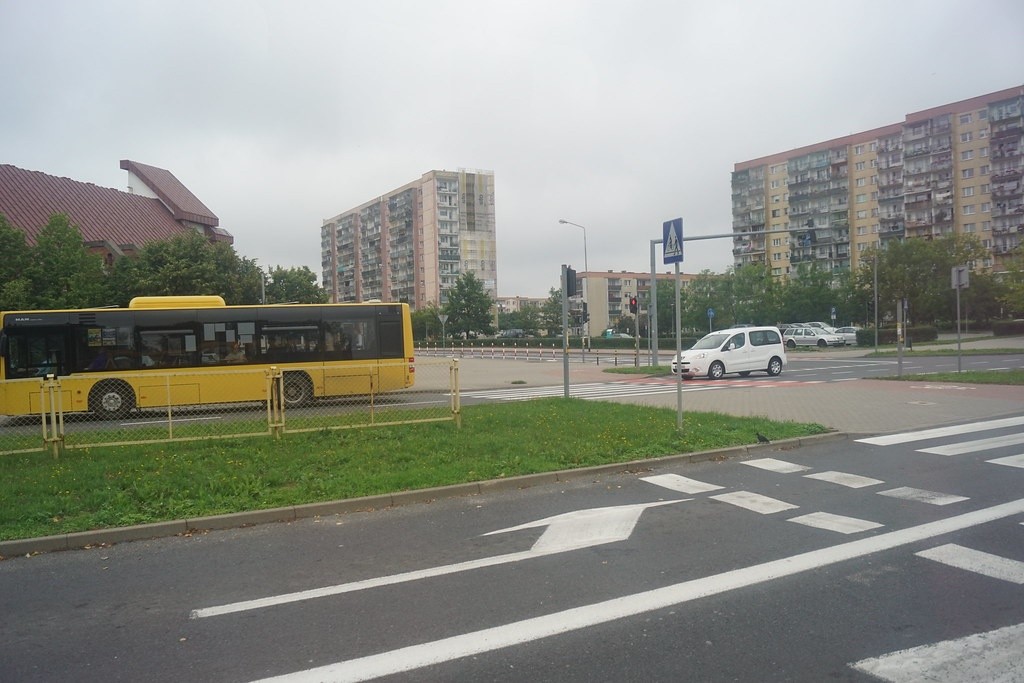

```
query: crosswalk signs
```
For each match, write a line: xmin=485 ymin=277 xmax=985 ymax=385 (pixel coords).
xmin=663 ymin=217 xmax=684 ymax=265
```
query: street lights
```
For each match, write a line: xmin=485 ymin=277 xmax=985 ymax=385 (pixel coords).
xmin=558 ymin=219 xmax=591 ymax=352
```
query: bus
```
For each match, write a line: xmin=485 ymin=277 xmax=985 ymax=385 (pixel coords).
xmin=0 ymin=295 xmax=415 ymax=419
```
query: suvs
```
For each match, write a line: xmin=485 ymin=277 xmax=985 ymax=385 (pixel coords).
xmin=782 ymin=327 xmax=846 ymax=347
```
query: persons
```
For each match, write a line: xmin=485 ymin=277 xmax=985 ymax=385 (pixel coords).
xmin=35 ymin=352 xmax=51 ymax=374
xmin=225 ymin=345 xmax=244 ymax=363
xmin=732 ymin=338 xmax=740 ymax=348
xmin=353 ymin=328 xmax=362 ymax=349
xmin=92 ymin=347 xmax=107 ymax=369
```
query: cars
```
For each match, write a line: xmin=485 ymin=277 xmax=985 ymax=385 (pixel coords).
xmin=831 ymin=326 xmax=864 ymax=345
xmin=778 ymin=322 xmax=837 ymax=335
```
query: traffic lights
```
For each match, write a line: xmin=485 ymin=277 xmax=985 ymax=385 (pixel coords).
xmin=630 ymin=298 xmax=637 ymax=315
xmin=868 ymin=301 xmax=875 ymax=317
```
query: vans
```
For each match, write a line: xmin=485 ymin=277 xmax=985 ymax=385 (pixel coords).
xmin=671 ymin=326 xmax=787 ymax=380
